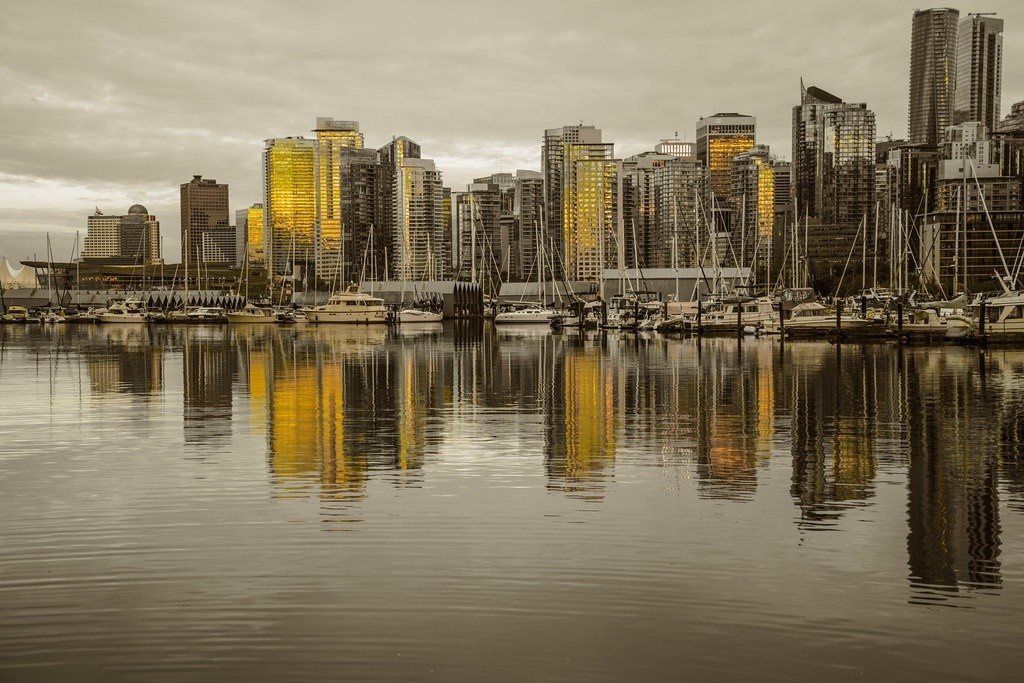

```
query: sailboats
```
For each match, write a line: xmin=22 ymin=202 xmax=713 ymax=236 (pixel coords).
xmin=0 ymin=148 xmax=1024 ymax=342
